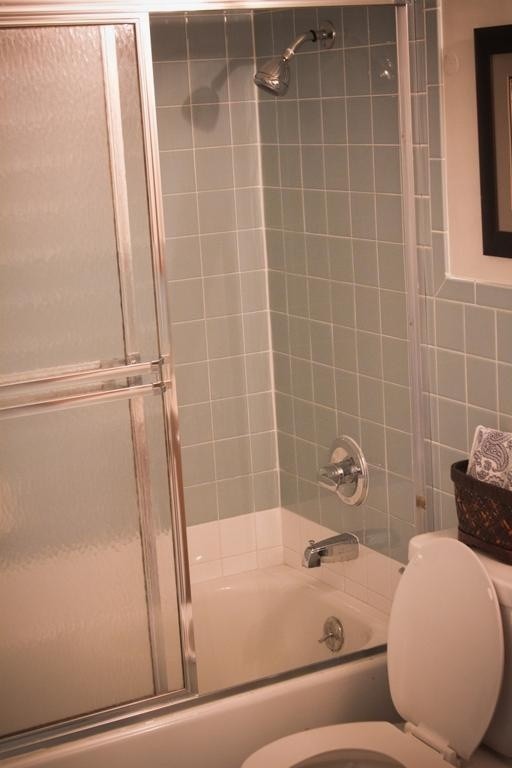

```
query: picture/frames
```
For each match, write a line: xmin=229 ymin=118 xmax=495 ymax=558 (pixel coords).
xmin=474 ymin=25 xmax=512 ymax=258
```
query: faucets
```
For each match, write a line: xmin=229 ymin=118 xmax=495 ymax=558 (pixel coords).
xmin=302 ymin=533 xmax=361 ymax=569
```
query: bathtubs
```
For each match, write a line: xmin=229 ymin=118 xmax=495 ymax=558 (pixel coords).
xmin=0 ymin=561 xmax=409 ymax=768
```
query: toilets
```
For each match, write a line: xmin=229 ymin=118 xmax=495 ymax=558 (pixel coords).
xmin=242 ymin=525 xmax=511 ymax=768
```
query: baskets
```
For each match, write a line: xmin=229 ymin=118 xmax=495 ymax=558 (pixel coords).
xmin=451 ymin=459 xmax=512 ymax=565
